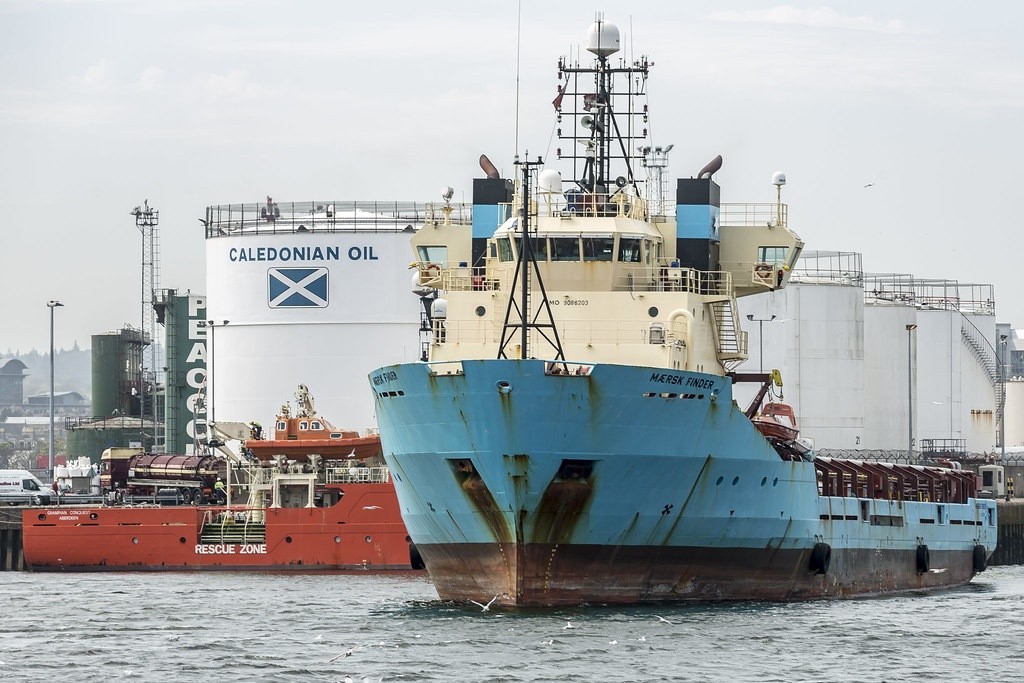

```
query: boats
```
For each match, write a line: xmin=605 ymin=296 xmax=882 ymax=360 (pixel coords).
xmin=748 ymin=402 xmax=801 ymax=444
xmin=244 ymin=381 xmax=386 ymax=464
xmin=789 ymin=435 xmax=815 ymax=456
xmin=370 ymin=11 xmax=1024 ymax=618
xmin=24 ymin=383 xmax=415 ymax=570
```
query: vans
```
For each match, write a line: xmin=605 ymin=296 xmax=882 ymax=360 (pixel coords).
xmin=0 ymin=469 xmax=57 ymax=505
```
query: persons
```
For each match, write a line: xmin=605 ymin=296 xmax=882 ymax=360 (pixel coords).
xmin=215 ymin=477 xmax=224 ymax=504
xmin=51 ymin=480 xmax=59 ymax=496
xmin=249 ymin=420 xmax=263 ymax=440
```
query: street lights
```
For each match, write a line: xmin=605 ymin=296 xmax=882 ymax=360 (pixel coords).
xmin=744 ymin=313 xmax=777 ymax=413
xmin=46 ymin=298 xmax=65 ymax=482
xmin=207 ymin=318 xmax=229 ymax=420
xmin=904 ymin=323 xmax=919 ymax=465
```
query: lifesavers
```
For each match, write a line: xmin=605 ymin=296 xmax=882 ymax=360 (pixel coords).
xmin=916 ymin=545 xmax=930 ymax=572
xmin=425 ymin=264 xmax=441 ymax=281
xmin=810 ymin=543 xmax=831 ymax=574
xmin=974 ymin=545 xmax=986 ymax=571
xmin=754 ymin=264 xmax=771 ymax=279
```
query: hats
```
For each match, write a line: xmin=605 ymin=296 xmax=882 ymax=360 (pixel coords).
xmin=217 ymin=478 xmax=221 ymax=480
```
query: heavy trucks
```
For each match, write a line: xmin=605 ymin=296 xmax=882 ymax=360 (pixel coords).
xmin=100 ymin=445 xmax=233 ymax=506
